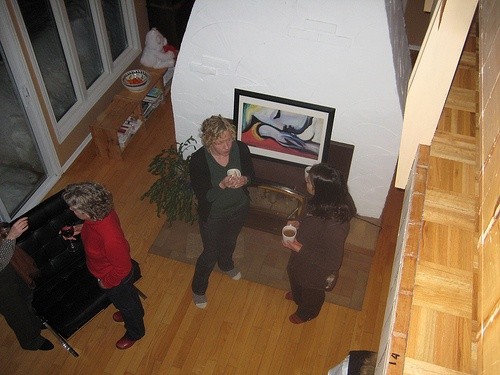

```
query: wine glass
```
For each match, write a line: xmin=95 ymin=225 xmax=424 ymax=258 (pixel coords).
xmin=61 ymin=223 xmax=80 ymax=253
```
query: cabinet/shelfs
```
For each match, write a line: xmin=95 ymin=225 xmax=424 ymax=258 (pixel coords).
xmin=89 ymin=65 xmax=174 ymax=161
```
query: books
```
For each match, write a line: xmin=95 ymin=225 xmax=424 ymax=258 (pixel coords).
xmin=118 ymin=115 xmax=143 ymax=150
xmin=142 ymin=86 xmax=164 ymax=119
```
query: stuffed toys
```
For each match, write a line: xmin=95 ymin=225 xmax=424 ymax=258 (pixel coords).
xmin=140 ymin=28 xmax=175 ymax=69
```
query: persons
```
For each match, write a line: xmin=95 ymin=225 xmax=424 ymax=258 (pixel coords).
xmin=189 ymin=114 xmax=254 ymax=309
xmin=0 ymin=217 xmax=54 ymax=351
xmin=58 ymin=183 xmax=145 ymax=350
xmin=280 ymin=163 xmax=358 ymax=324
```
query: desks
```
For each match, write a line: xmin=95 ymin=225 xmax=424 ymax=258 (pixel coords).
xmin=246 ymin=140 xmax=355 ymax=236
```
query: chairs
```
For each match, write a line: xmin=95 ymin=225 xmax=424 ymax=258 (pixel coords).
xmin=7 ymin=190 xmax=147 ymax=356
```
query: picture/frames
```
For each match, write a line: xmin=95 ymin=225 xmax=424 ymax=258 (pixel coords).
xmin=233 ymin=89 xmax=336 ymax=167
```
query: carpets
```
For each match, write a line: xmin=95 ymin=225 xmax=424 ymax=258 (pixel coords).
xmin=150 ymin=200 xmax=382 ymax=311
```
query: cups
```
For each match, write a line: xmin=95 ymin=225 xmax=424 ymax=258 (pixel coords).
xmin=282 ymin=225 xmax=297 ymax=244
xmin=227 ymin=169 xmax=241 ymax=178
xmin=0 ymin=222 xmax=10 ymax=239
xmin=304 ymin=167 xmax=312 ymax=182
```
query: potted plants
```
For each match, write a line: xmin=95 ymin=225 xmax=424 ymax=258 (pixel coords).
xmin=141 ymin=141 xmax=202 ymax=227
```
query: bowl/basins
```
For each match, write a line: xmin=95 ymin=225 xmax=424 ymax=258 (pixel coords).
xmin=120 ymin=69 xmax=151 ymax=92
